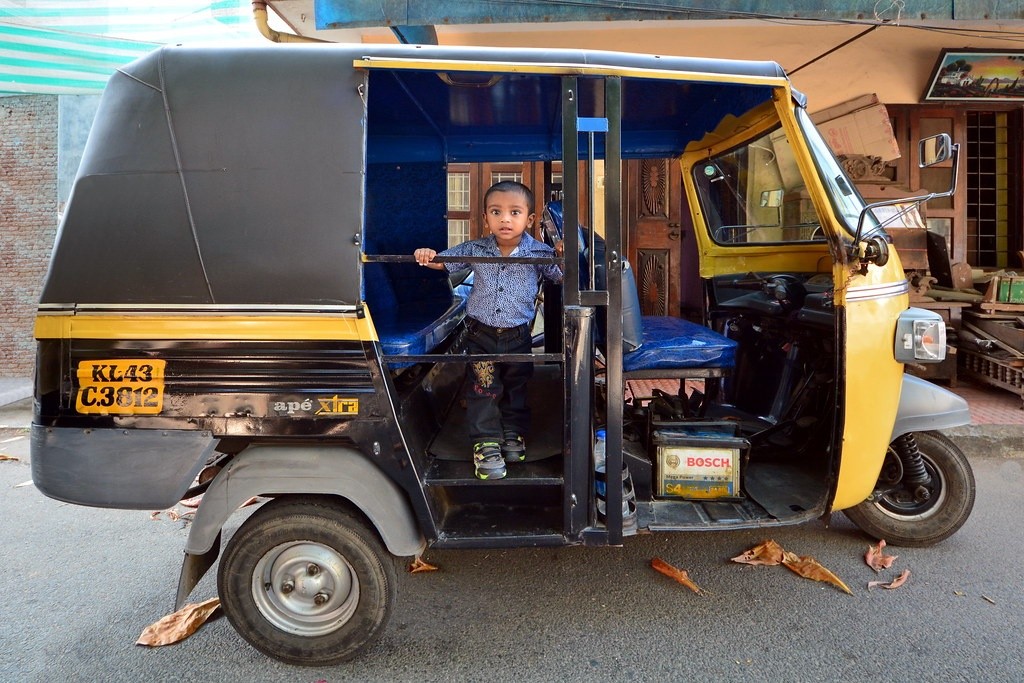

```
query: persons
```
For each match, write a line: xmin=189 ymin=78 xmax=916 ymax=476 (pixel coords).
xmin=414 ymin=181 xmax=563 ymax=480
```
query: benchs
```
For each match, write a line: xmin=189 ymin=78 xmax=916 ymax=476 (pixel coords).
xmin=542 ymin=199 xmax=738 ymax=379
xmin=365 ymin=241 xmax=467 ymax=369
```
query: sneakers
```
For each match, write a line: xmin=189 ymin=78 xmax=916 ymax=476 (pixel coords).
xmin=500 ymin=431 xmax=525 ymax=462
xmin=473 ymin=440 xmax=506 ymax=479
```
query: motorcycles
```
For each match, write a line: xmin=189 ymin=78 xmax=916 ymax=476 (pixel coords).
xmin=29 ymin=44 xmax=976 ymax=666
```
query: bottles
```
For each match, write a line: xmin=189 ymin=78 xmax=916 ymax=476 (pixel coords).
xmin=593 ymin=430 xmax=629 ymax=518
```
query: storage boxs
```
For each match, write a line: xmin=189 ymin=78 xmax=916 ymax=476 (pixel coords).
xmin=972 ymin=275 xmax=1024 ymax=304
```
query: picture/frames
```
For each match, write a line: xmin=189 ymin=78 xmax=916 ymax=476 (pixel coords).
xmin=918 ymin=48 xmax=1024 ymax=103
